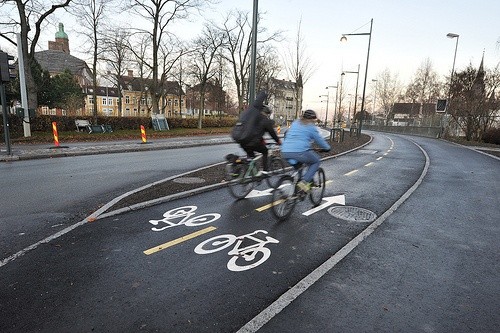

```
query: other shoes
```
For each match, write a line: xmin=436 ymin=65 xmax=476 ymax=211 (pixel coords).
xmin=296 ymin=181 xmax=314 ymax=194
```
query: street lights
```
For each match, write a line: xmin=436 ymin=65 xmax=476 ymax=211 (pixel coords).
xmin=318 ymin=79 xmax=352 ymax=128
xmin=371 ymin=79 xmax=378 ymax=125
xmin=339 ymin=17 xmax=374 ymax=139
xmin=340 ymin=64 xmax=360 ymax=136
xmin=438 ymin=32 xmax=460 ymax=138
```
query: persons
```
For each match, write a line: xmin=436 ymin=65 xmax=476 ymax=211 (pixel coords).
xmin=240 ymin=104 xmax=282 ymax=182
xmin=280 ymin=110 xmax=330 ymax=194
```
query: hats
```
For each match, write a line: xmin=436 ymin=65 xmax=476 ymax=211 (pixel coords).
xmin=303 ymin=109 xmax=317 ymax=119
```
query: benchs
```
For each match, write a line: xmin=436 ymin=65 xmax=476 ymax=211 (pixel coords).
xmin=75 ymin=119 xmax=91 ymax=132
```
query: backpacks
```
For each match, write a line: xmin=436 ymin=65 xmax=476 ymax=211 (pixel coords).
xmin=232 ymin=109 xmax=258 ymax=144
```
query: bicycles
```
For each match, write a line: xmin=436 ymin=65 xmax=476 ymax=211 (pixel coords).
xmin=269 ymin=147 xmax=332 ymax=221
xmin=224 ymin=141 xmax=286 ymax=200
